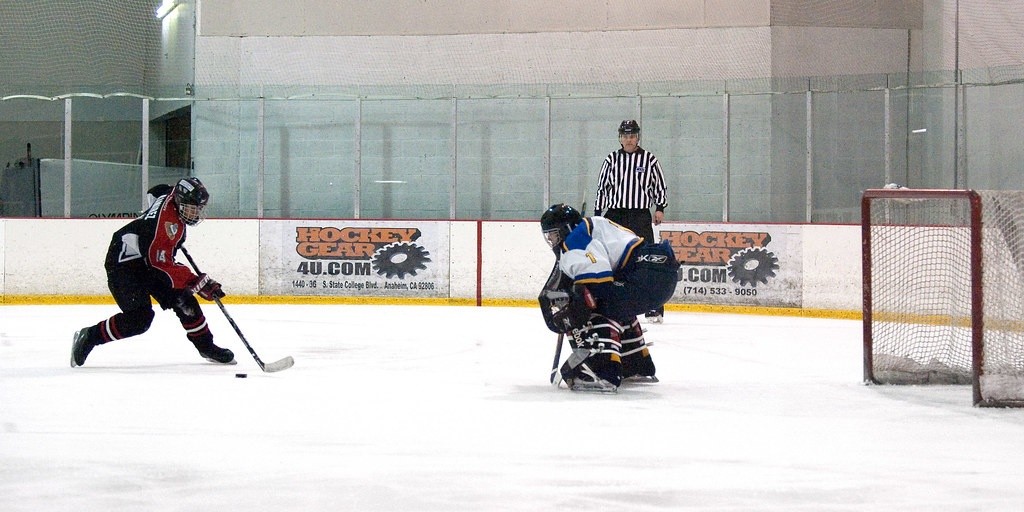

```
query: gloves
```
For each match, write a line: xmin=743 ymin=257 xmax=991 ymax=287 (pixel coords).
xmin=192 ymin=273 xmax=226 ymax=301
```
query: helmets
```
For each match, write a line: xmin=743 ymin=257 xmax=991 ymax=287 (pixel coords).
xmin=174 ymin=177 xmax=209 ymax=226
xmin=618 ymin=119 xmax=640 ymax=134
xmin=540 ymin=203 xmax=582 ymax=260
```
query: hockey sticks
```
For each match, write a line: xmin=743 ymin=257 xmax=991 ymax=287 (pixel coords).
xmin=176 ymin=243 xmax=295 ymax=373
xmin=547 ymin=194 xmax=591 ymax=392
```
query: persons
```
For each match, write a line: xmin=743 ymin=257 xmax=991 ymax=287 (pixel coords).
xmin=70 ymin=177 xmax=237 ymax=368
xmin=538 ymin=203 xmax=678 ymax=395
xmin=594 ymin=120 xmax=668 ymax=323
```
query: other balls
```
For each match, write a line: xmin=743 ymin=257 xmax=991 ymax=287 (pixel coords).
xmin=234 ymin=373 xmax=248 ymax=378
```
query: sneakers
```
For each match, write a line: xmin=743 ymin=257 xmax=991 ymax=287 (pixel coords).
xmin=70 ymin=326 xmax=94 ymax=368
xmin=569 ymin=370 xmax=622 ymax=395
xmin=644 ymin=308 xmax=664 ymax=324
xmin=620 ymin=362 xmax=659 ymax=382
xmin=198 ymin=344 xmax=238 ymax=365
